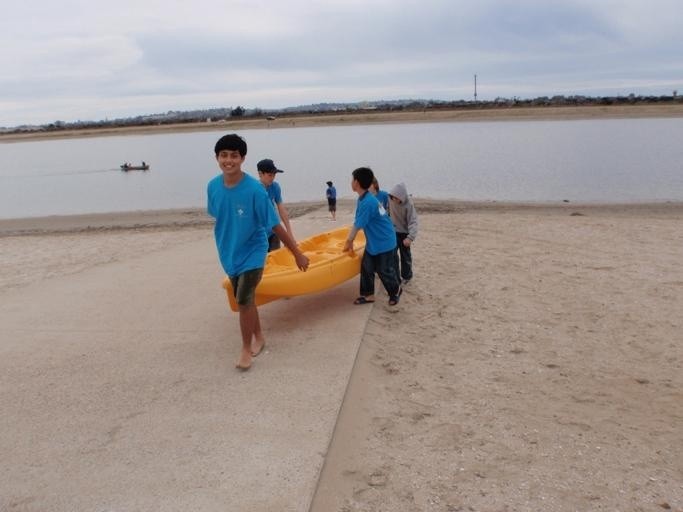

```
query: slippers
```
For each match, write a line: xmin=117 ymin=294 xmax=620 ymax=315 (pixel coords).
xmin=355 ymin=296 xmax=374 ymax=305
xmin=389 ymin=288 xmax=403 ymax=305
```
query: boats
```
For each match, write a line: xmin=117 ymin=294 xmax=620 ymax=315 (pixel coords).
xmin=118 ymin=160 xmax=149 ymax=173
xmin=221 ymin=225 xmax=367 ymax=312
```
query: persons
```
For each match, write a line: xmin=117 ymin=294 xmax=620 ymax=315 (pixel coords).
xmin=206 ymin=133 xmax=310 ymax=373
xmin=366 ymin=175 xmax=388 ymax=213
xmin=254 ymin=158 xmax=299 ymax=253
xmin=326 ymin=181 xmax=336 ymax=221
xmin=384 ymin=183 xmax=418 ymax=286
xmin=341 ymin=166 xmax=404 ymax=306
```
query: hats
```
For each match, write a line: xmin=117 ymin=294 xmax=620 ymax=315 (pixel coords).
xmin=257 ymin=159 xmax=284 ymax=173
